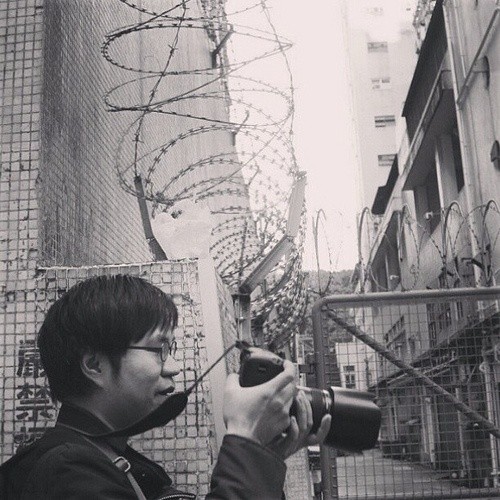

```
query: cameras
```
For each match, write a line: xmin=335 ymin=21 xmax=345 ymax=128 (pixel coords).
xmin=237 ymin=339 xmax=384 ymax=455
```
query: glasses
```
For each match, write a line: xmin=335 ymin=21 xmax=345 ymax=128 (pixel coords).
xmin=128 ymin=341 xmax=177 ymax=362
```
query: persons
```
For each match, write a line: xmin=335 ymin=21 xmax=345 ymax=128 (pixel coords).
xmin=1 ymin=273 xmax=332 ymax=500
